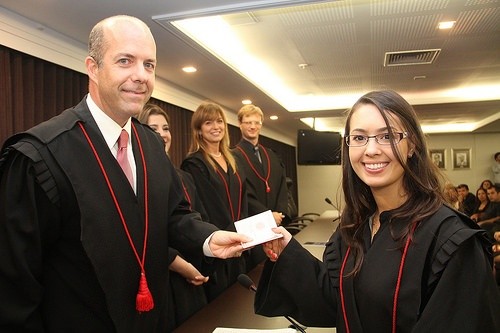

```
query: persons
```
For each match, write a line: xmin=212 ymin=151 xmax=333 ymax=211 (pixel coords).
xmin=253 ymin=89 xmax=500 ymax=333
xmin=444 ymin=152 xmax=500 ymax=282
xmin=179 ymin=101 xmax=248 ymax=300
xmin=136 ymin=103 xmax=210 ymax=325
xmin=230 ymin=104 xmax=289 ymax=227
xmin=0 ymin=14 xmax=252 ymax=333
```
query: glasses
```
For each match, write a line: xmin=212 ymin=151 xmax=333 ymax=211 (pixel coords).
xmin=345 ymin=132 xmax=410 ymax=147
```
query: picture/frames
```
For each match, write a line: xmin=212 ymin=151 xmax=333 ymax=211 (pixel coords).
xmin=430 ymin=146 xmax=471 ymax=172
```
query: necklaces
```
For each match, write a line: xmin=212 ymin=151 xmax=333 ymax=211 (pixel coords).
xmin=210 ymin=151 xmax=222 ymax=158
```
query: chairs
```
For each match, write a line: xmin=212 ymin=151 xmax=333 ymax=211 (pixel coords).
xmin=287 ymin=212 xmax=321 ymax=231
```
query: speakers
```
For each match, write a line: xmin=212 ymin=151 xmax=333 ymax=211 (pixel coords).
xmin=298 ymin=130 xmax=342 ymax=166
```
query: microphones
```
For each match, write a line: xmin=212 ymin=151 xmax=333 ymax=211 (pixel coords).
xmin=325 ymin=198 xmax=342 ymax=214
xmin=237 ymin=273 xmax=307 ymax=333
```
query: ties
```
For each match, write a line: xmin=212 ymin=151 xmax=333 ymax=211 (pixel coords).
xmin=255 ymin=146 xmax=260 ymax=162
xmin=117 ymin=129 xmax=134 ymax=192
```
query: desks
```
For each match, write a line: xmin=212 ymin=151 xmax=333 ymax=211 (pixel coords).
xmin=177 ymin=210 xmax=342 ymax=330
